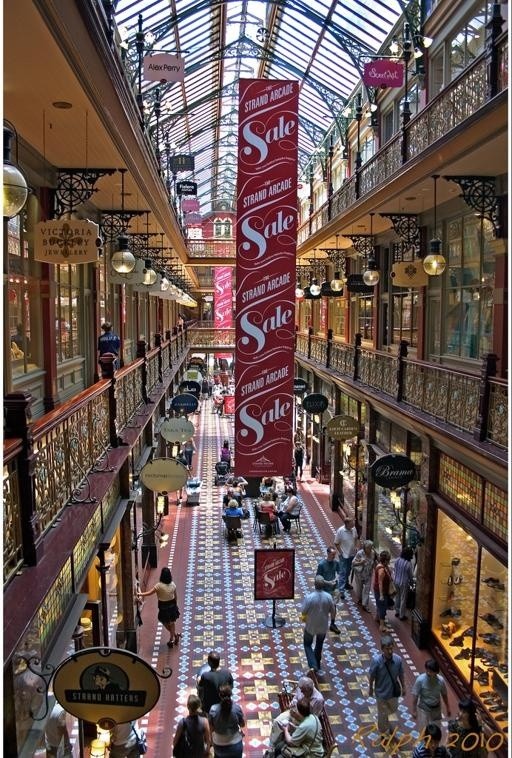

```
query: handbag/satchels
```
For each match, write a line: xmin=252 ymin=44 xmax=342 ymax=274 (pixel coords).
xmin=138 ymin=729 xmax=148 ymax=754
xmin=393 ymin=681 xmax=400 ymax=697
xmin=388 ymin=581 xmax=397 ymax=595
xmin=173 ymin=728 xmax=193 ymax=758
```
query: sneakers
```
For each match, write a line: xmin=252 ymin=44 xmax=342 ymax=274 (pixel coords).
xmin=330 ymin=625 xmax=340 ymax=633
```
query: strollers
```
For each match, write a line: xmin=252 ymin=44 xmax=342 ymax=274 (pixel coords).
xmin=215 ymin=461 xmax=230 ymax=484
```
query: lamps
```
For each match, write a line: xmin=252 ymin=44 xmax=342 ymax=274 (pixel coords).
xmin=129 ymin=495 xmax=173 ymax=556
xmin=70 ymin=718 xmax=124 ymax=758
xmin=5 ymin=116 xmax=201 ymax=312
xmin=295 ymin=176 xmax=450 ymax=300
xmin=169 ymin=439 xmax=189 ymax=461
xmin=293 ymin=390 xmax=422 ymax=542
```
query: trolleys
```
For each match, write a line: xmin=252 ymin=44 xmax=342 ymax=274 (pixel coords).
xmin=186 ymin=477 xmax=202 ymax=506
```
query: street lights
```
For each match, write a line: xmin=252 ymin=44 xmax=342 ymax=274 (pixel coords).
xmin=119 ymin=13 xmax=156 ymax=113
xmin=316 ymin=133 xmax=345 ymax=224
xmin=302 ymin=157 xmax=323 ymax=236
xmin=163 ymin=167 xmax=182 ymax=206
xmin=342 ymin=91 xmax=378 ymax=199
xmin=142 ymin=87 xmax=171 ymax=167
xmin=389 ymin=21 xmax=434 ymax=165
xmin=156 ymin=131 xmax=179 ymax=195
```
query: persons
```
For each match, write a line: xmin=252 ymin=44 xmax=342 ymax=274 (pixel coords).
xmin=97 ymin=321 xmax=121 ymax=372
xmin=12 ymin=318 xmax=70 ymax=352
xmin=45 ymin=704 xmax=73 ymax=757
xmin=175 ymin=310 xmax=212 ymax=328
xmin=106 ymin=359 xmax=488 ymax=757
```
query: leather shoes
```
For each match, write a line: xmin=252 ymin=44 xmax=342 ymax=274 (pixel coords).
xmin=479 ymin=613 xmax=503 ymax=629
xmin=481 ymin=577 xmax=504 ymax=590
xmin=450 ymin=626 xmax=508 ymax=732
